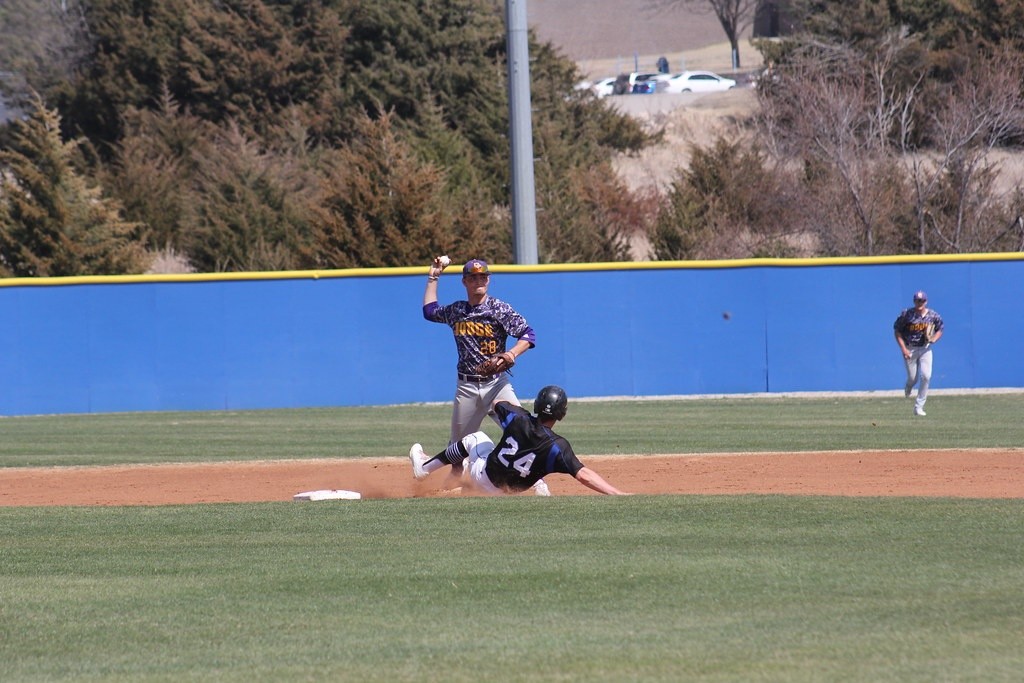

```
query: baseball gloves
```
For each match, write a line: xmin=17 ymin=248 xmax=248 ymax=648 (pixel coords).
xmin=474 ymin=353 xmax=515 ymax=375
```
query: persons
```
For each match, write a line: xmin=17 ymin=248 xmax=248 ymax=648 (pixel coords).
xmin=894 ymin=290 xmax=944 ymax=416
xmin=408 ymin=386 xmax=634 ymax=496
xmin=423 ymin=256 xmax=554 ymax=496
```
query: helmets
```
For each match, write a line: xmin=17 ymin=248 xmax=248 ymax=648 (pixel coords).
xmin=534 ymin=385 xmax=568 ymax=422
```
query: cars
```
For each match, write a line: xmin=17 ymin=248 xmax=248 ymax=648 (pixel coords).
xmin=589 ymin=72 xmax=677 ymax=100
xmin=655 ymin=71 xmax=735 ymax=94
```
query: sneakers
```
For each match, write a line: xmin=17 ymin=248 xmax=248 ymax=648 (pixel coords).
xmin=409 ymin=442 xmax=430 ymax=483
xmin=530 ymin=478 xmax=551 ymax=497
xmin=442 ymin=467 xmax=463 ymax=489
xmin=460 ymin=456 xmax=474 ymax=487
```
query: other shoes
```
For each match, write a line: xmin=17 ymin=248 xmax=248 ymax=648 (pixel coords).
xmin=905 ymin=387 xmax=911 ymax=399
xmin=913 ymin=408 xmax=927 ymax=416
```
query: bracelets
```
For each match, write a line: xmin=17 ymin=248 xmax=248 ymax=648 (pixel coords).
xmin=428 ymin=275 xmax=439 ymax=280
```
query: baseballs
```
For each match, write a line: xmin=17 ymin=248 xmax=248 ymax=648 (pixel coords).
xmin=439 ymin=256 xmax=449 ymax=266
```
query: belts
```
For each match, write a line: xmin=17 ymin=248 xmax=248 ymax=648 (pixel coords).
xmin=457 ymin=373 xmax=499 ymax=382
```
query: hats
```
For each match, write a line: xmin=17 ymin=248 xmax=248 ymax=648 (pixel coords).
xmin=914 ymin=290 xmax=927 ymax=301
xmin=463 ymin=259 xmax=491 ymax=279
